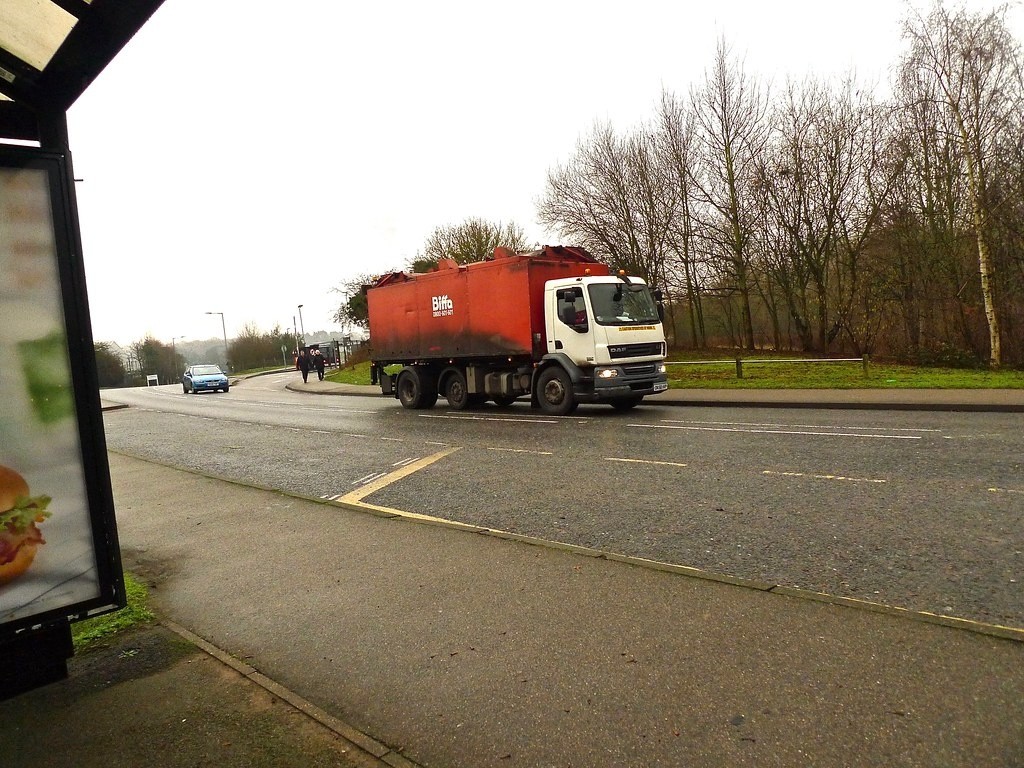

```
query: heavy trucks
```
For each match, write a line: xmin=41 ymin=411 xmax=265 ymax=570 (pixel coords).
xmin=361 ymin=245 xmax=668 ymax=417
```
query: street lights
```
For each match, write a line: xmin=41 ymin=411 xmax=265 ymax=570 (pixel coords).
xmin=205 ymin=311 xmax=228 ymax=360
xmin=297 ymin=304 xmax=305 ymax=344
xmin=172 ymin=336 xmax=183 ymax=370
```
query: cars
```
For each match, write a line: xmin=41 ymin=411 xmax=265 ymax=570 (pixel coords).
xmin=183 ymin=365 xmax=230 ymax=394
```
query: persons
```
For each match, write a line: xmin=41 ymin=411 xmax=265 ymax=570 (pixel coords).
xmin=296 ymin=351 xmax=313 ymax=383
xmin=312 ymin=350 xmax=331 ymax=381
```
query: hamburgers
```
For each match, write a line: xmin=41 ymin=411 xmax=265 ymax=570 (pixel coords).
xmin=0 ymin=464 xmax=53 ymax=585
